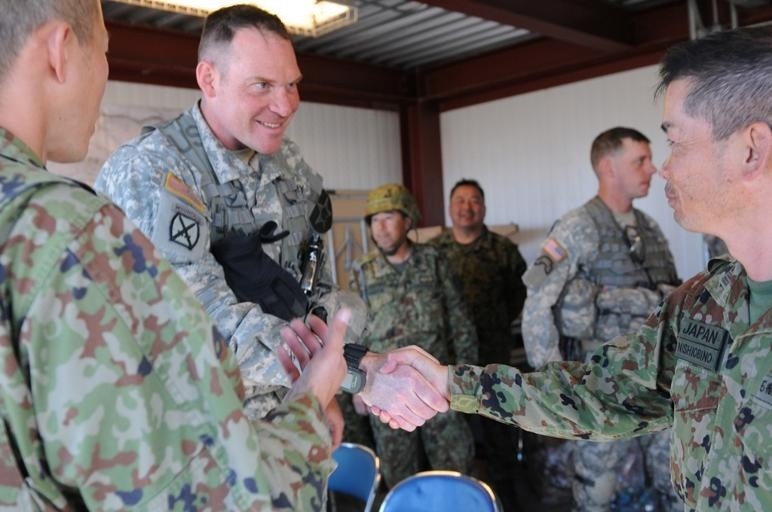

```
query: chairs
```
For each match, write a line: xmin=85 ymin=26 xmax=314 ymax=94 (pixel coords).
xmin=327 ymin=442 xmax=507 ymax=512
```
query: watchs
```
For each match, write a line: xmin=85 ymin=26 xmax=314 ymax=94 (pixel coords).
xmin=338 ymin=341 xmax=369 ymax=398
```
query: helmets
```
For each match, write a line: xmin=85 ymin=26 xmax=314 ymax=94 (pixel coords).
xmin=361 ymin=182 xmax=421 ymax=224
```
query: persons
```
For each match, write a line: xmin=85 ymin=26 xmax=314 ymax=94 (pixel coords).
xmin=520 ymin=125 xmax=685 ymax=511
xmin=356 ymin=28 xmax=772 ymax=511
xmin=0 ymin=1 xmax=353 ymax=511
xmin=96 ymin=4 xmax=448 ymax=511
xmin=421 ymin=176 xmax=529 ymax=511
xmin=344 ymin=182 xmax=482 ymax=492
